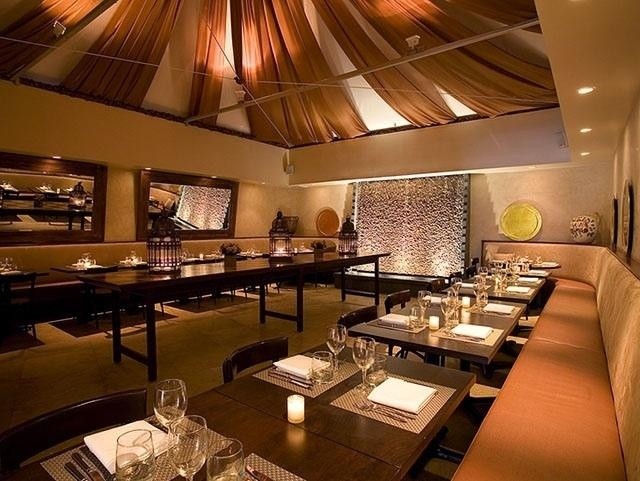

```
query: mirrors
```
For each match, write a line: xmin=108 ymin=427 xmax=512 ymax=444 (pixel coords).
xmin=136 ymin=170 xmax=239 ymax=242
xmin=0 ymin=151 xmax=108 ymax=243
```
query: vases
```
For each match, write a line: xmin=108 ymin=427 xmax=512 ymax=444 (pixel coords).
xmin=570 ymin=215 xmax=599 ymax=244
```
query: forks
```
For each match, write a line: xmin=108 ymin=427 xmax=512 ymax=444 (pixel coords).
xmin=350 ymin=398 xmax=416 ymax=424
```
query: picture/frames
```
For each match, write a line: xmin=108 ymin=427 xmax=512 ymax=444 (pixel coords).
xmin=608 ymin=197 xmax=618 ymax=244
xmin=621 ymin=179 xmax=634 ymax=255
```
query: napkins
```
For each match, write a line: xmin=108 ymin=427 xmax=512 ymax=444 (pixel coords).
xmin=364 ymin=377 xmax=438 ymax=414
xmin=81 ymin=417 xmax=181 ymax=475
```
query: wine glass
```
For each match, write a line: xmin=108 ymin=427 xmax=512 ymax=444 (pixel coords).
xmin=413 ymin=256 xmax=530 ymax=338
xmin=325 ymin=323 xmax=348 ymax=372
xmin=351 ymin=336 xmax=376 ymax=393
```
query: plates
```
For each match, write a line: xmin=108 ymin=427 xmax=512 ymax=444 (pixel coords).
xmin=315 ymin=207 xmax=338 ymax=236
xmin=499 ymin=203 xmax=543 ymax=241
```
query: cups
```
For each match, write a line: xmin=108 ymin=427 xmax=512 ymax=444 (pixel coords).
xmin=311 ymin=351 xmax=333 ymax=385
xmin=113 ymin=379 xmax=245 ymax=481
xmin=365 ymin=353 xmax=385 ymax=385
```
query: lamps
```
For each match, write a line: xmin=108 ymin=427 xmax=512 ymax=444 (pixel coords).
xmin=405 ymin=34 xmax=420 ymax=55
xmin=52 ymin=19 xmax=66 ymax=39
xmin=234 ymin=84 xmax=246 ymax=106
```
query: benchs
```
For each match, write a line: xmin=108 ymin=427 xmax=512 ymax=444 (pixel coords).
xmin=448 ymin=237 xmax=640 ymax=480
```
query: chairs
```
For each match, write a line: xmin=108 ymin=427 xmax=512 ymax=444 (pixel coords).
xmin=0 ymin=179 xmax=93 ymax=231
xmin=2 ymin=387 xmax=149 ymax=475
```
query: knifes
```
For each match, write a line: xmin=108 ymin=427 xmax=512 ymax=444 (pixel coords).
xmin=63 ymin=452 xmax=104 ymax=481
xmin=245 ymin=462 xmax=273 ymax=481
xmin=265 ymin=368 xmax=313 ymax=390
